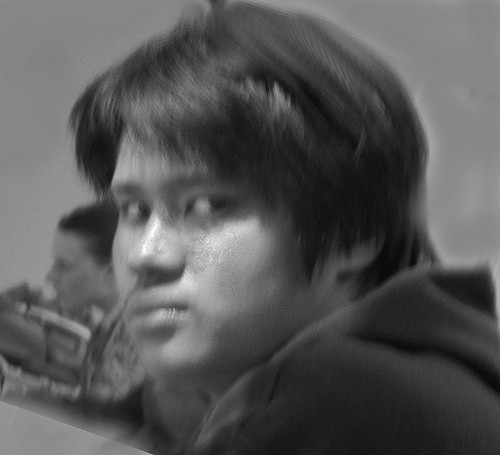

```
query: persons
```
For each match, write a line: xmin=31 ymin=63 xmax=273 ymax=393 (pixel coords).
xmin=65 ymin=3 xmax=499 ymax=455
xmin=0 ymin=198 xmax=149 ymax=430
